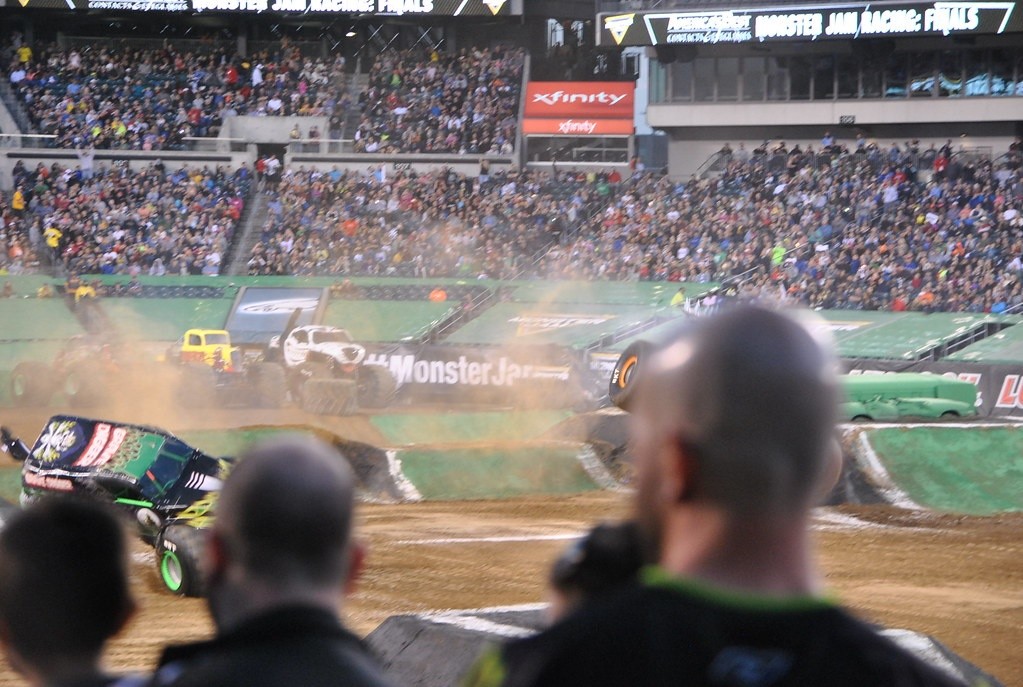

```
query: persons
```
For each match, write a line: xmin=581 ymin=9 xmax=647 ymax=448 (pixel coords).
xmin=0 ymin=489 xmax=154 ymax=687
xmin=0 ymin=34 xmax=1023 ymax=314
xmin=152 ymin=433 xmax=397 ymax=687
xmin=546 ymin=493 xmax=655 ymax=622
xmin=461 ymin=303 xmax=968 ymax=687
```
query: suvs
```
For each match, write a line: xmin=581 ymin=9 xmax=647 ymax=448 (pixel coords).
xmin=249 ymin=324 xmax=395 ymax=416
xmin=545 ymin=339 xmax=655 ymax=492
xmin=21 ymin=413 xmax=240 ymax=599
xmin=160 ymin=328 xmax=258 ymax=410
xmin=9 ymin=333 xmax=168 ymax=420
